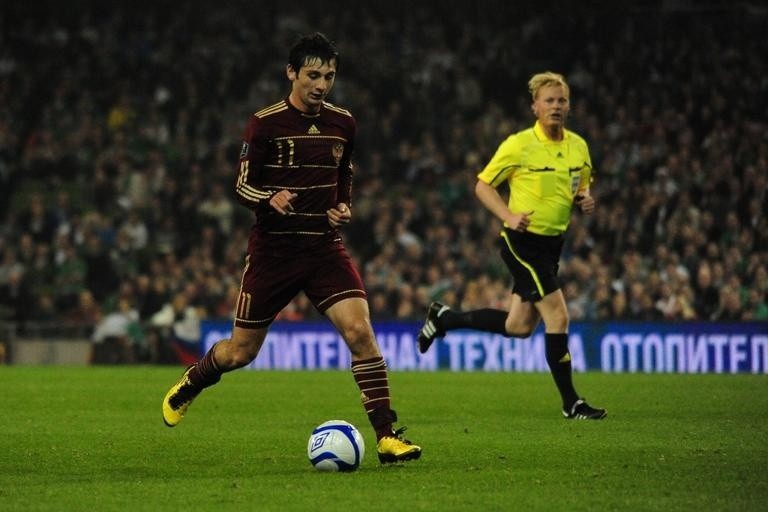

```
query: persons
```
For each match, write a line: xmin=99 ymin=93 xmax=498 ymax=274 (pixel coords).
xmin=1 ymin=1 xmax=768 ymax=322
xmin=149 ymin=295 xmax=199 ymax=362
xmin=160 ymin=32 xmax=424 ymax=463
xmin=91 ymin=308 xmax=140 ymax=361
xmin=416 ymin=71 xmax=609 ymax=420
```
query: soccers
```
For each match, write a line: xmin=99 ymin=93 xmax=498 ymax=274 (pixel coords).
xmin=307 ymin=420 xmax=365 ymax=471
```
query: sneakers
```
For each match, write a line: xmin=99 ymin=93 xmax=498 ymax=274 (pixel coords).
xmin=161 ymin=363 xmax=201 ymax=428
xmin=419 ymin=300 xmax=451 ymax=353
xmin=563 ymin=397 xmax=607 ymax=419
xmin=377 ymin=432 xmax=420 ymax=463
